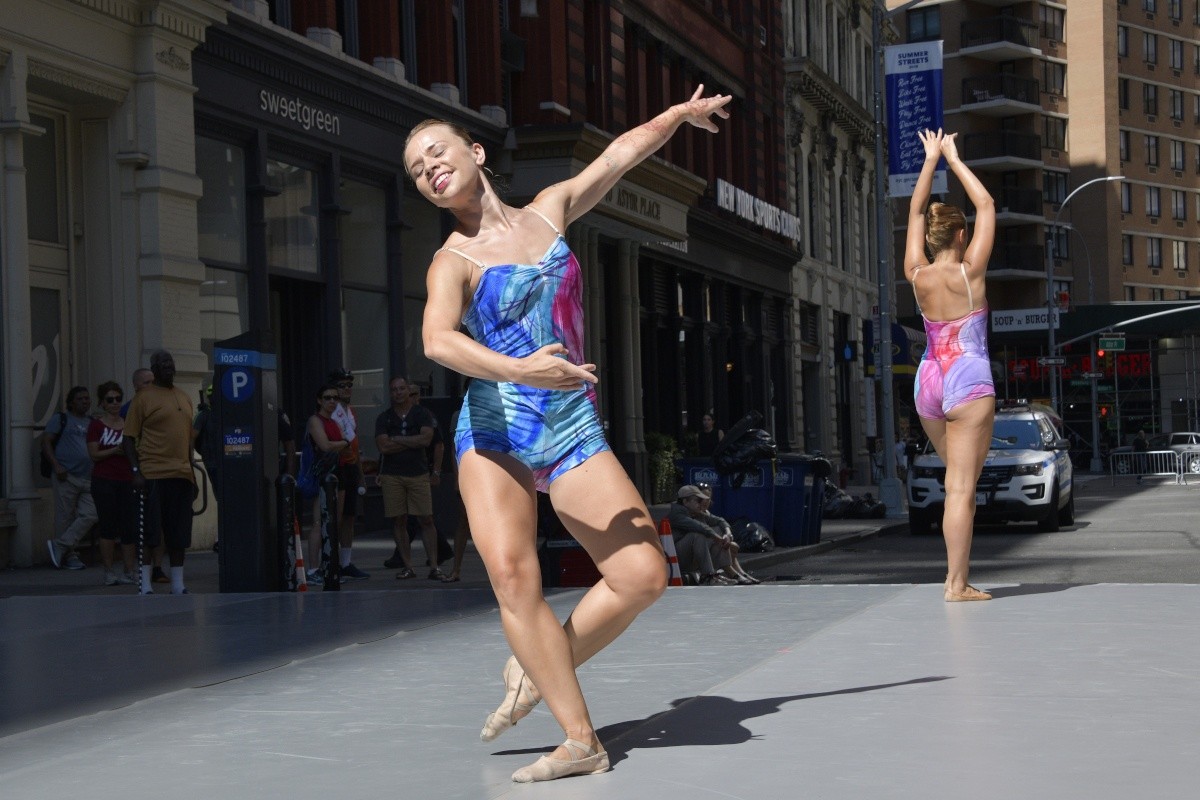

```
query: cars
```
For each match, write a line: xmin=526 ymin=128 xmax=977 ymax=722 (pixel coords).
xmin=1105 ymin=432 xmax=1200 ymax=475
xmin=904 ymin=399 xmax=1076 ymax=534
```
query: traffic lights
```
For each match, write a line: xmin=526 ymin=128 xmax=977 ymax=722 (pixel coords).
xmin=1097 ymin=406 xmax=1113 ymax=418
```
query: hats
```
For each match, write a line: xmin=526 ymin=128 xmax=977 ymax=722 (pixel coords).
xmin=678 ymin=485 xmax=710 ymax=499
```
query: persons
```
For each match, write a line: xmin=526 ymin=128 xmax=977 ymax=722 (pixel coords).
xmin=693 ymin=413 xmax=725 ymax=459
xmin=667 ymin=482 xmax=760 ymax=586
xmin=903 ymin=128 xmax=997 ymax=601
xmin=39 ymin=349 xmax=471 ymax=595
xmin=402 ymin=82 xmax=733 ymax=784
xmin=1130 ymin=430 xmax=1150 ymax=484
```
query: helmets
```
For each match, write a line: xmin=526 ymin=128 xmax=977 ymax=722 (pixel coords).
xmin=334 ymin=368 xmax=354 ymax=382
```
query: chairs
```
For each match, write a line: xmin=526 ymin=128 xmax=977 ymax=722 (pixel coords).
xmin=972 ymin=89 xmax=1005 ymax=103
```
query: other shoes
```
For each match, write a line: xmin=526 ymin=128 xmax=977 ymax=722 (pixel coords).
xmin=428 ymin=569 xmax=442 ymax=579
xmin=441 ymin=576 xmax=461 ymax=583
xmin=733 ymin=569 xmax=760 ymax=584
xmin=120 ymin=572 xmax=138 ymax=584
xmin=384 ymin=558 xmax=405 ymax=568
xmin=698 ymin=573 xmax=738 ymax=586
xmin=47 ymin=538 xmax=66 ymax=568
xmin=396 ymin=567 xmax=416 ymax=579
xmin=338 ymin=562 xmax=370 ymax=579
xmin=305 ymin=569 xmax=346 ymax=585
xmin=426 ymin=550 xmax=454 ymax=567
xmin=105 ymin=572 xmax=120 ymax=585
xmin=62 ymin=555 xmax=85 ymax=570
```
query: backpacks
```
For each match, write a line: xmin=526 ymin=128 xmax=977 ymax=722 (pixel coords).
xmin=41 ymin=412 xmax=95 ymax=478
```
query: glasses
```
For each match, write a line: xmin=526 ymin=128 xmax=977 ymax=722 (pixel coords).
xmin=323 ymin=395 xmax=339 ymax=401
xmin=409 ymin=393 xmax=418 ymax=397
xmin=105 ymin=396 xmax=122 ymax=403
xmin=336 ymin=383 xmax=353 ymax=388
xmin=698 ymin=483 xmax=708 ymax=488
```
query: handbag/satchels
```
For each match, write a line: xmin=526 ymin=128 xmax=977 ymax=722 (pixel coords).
xmin=297 ymin=434 xmax=319 ymax=499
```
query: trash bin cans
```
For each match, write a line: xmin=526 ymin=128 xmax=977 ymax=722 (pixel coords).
xmin=681 ymin=455 xmax=828 ymax=546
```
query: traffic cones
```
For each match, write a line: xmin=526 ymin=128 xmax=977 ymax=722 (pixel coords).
xmin=659 ymin=517 xmax=683 ymax=587
xmin=295 ymin=520 xmax=310 ymax=592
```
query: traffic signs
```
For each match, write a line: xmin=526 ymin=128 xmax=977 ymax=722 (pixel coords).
xmin=1036 ymin=358 xmax=1067 ymax=366
xmin=1079 ymin=370 xmax=1106 ymax=382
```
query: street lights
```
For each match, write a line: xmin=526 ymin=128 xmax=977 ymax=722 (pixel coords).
xmin=1048 ymin=175 xmax=1128 ymax=405
xmin=1062 ymin=225 xmax=1104 ymax=472
